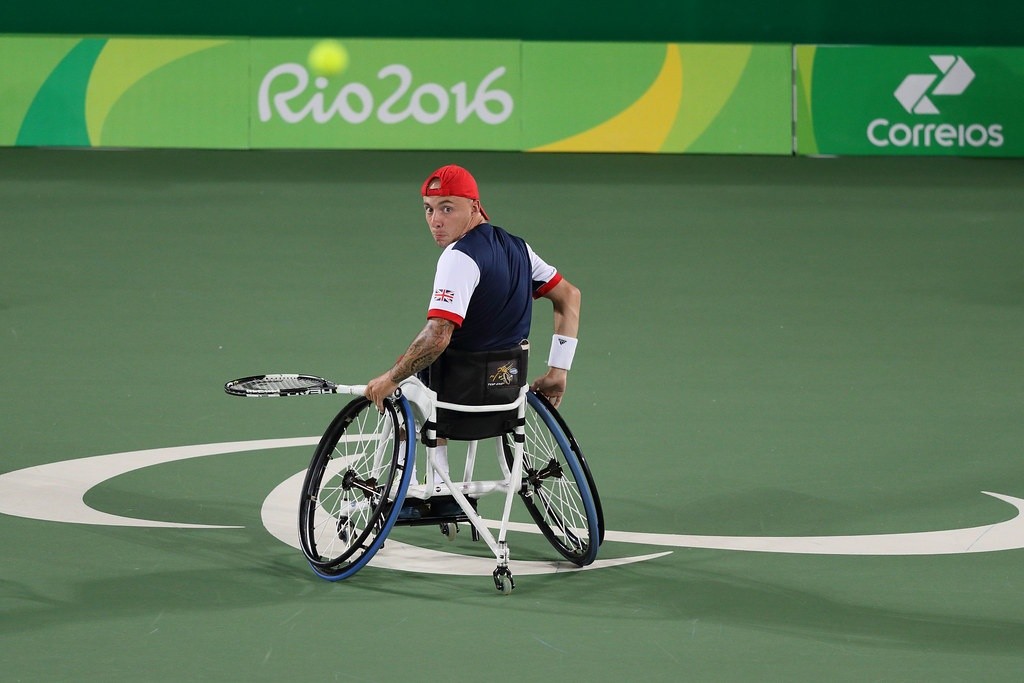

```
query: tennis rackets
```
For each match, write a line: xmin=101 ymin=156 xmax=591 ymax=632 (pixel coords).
xmin=225 ymin=373 xmax=404 ymax=400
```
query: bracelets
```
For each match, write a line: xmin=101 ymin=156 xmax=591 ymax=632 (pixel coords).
xmin=548 ymin=334 xmax=579 ymax=371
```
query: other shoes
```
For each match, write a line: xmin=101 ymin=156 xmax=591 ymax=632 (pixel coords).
xmin=422 ymin=471 xmax=451 ymax=486
xmin=384 ymin=475 xmax=420 ymax=499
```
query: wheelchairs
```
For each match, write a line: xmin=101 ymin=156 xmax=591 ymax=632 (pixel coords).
xmin=298 ymin=339 xmax=605 ymax=597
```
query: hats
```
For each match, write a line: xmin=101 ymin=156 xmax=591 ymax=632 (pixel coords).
xmin=421 ymin=164 xmax=490 ymax=221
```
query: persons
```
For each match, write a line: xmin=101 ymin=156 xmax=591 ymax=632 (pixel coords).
xmin=365 ymin=164 xmax=580 ymax=489
